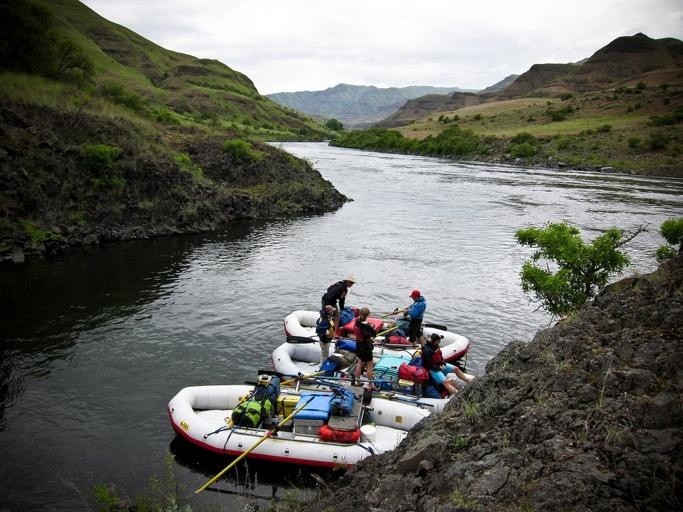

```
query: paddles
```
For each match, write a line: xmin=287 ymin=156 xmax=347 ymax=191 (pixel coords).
xmin=258 ymin=370 xmax=394 ymax=383
xmin=243 ymin=380 xmax=333 ymax=392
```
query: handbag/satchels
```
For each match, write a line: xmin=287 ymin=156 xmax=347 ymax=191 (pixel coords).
xmin=255 ymin=375 xmax=281 ymax=401
xmin=335 ymin=339 xmax=356 ymax=353
xmin=340 ymin=306 xmax=354 ymax=325
xmin=387 ymin=320 xmax=410 ymax=338
xmin=328 ymin=387 xmax=354 ymax=416
xmin=398 ymin=362 xmax=430 ymax=384
xmin=329 ymin=350 xmax=357 ymax=368
xmin=385 ymin=336 xmax=410 ymax=344
xmin=232 ymin=397 xmax=272 ymax=429
xmin=319 ymin=424 xmax=361 ymax=443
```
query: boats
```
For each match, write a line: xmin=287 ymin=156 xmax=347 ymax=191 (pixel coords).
xmin=164 ymin=383 xmax=431 ymax=474
xmin=269 ymin=338 xmax=476 ymax=414
xmin=282 ymin=309 xmax=469 ymax=364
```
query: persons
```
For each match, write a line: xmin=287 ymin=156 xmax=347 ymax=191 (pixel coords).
xmin=315 ymin=305 xmax=336 ymax=368
xmin=321 ymin=277 xmax=356 ymax=312
xmin=353 ymin=307 xmax=378 ymax=391
xmin=420 ymin=332 xmax=472 ymax=394
xmin=402 ymin=289 xmax=426 ymax=349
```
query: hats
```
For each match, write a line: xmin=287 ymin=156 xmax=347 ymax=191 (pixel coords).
xmin=343 ymin=276 xmax=357 ymax=283
xmin=409 ymin=290 xmax=420 ymax=298
xmin=325 ymin=305 xmax=336 ymax=313
xmin=431 ymin=333 xmax=444 ymax=341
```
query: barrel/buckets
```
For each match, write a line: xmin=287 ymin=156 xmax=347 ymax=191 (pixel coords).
xmin=360 ymin=424 xmax=376 ymax=444
